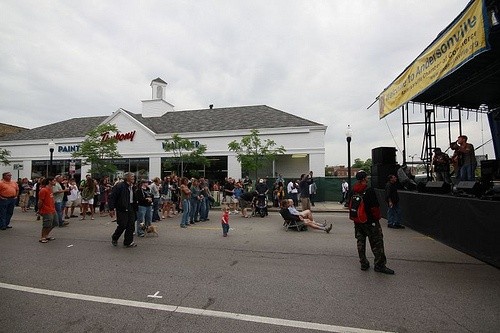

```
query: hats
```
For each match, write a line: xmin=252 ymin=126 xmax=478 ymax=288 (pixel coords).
xmin=356 ymin=172 xmax=369 ymax=180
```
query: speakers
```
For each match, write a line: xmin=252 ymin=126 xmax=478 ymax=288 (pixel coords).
xmin=424 ymin=181 xmax=452 ymax=194
xmin=371 ymin=176 xmax=398 ymax=189
xmin=456 ymin=181 xmax=487 ymax=198
xmin=370 ymin=165 xmax=396 ymax=176
xmin=371 ymin=147 xmax=396 ymax=164
xmin=398 ymin=168 xmax=417 ymax=191
xmin=480 ymin=159 xmax=500 ymax=181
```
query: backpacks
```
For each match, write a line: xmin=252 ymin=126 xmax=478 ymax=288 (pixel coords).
xmin=349 ymin=187 xmax=367 ymax=224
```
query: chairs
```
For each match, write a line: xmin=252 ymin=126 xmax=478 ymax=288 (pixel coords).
xmin=278 ymin=210 xmax=303 ymax=232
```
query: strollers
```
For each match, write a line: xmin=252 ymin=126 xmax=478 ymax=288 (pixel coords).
xmin=252 ymin=194 xmax=267 ymax=218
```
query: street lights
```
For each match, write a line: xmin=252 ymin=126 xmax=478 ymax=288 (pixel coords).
xmin=409 ymin=153 xmax=417 ymax=169
xmin=345 ymin=124 xmax=353 ymax=207
xmin=48 ymin=136 xmax=55 ymax=182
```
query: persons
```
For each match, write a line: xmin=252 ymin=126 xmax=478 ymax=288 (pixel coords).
xmin=338 ymin=180 xmax=348 ymax=208
xmin=347 ymin=169 xmax=395 ymax=274
xmin=432 ymin=135 xmax=478 ymax=183
xmin=0 ymin=169 xmax=333 ymax=248
xmin=384 ymin=174 xmax=406 ymax=229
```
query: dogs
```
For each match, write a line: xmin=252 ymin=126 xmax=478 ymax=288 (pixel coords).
xmin=137 ymin=222 xmax=160 ymax=240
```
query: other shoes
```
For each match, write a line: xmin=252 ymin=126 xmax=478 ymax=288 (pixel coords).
xmin=325 ymin=224 xmax=332 ymax=233
xmin=128 ymin=241 xmax=137 ymax=247
xmin=180 ymin=219 xmax=210 ymax=228
xmin=323 ymin=219 xmax=327 ymax=227
xmin=65 ymin=215 xmax=70 ymax=219
xmin=70 ymin=215 xmax=78 ymax=218
xmin=361 ymin=265 xmax=370 ymax=270
xmin=373 ymin=265 xmax=395 ymax=274
xmin=387 ymin=223 xmax=405 ymax=229
xmin=39 ymin=236 xmax=55 ymax=243
xmin=112 ymin=239 xmax=117 ymax=246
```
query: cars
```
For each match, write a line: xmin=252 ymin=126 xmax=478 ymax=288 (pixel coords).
xmin=413 ymin=172 xmax=433 ymax=178
